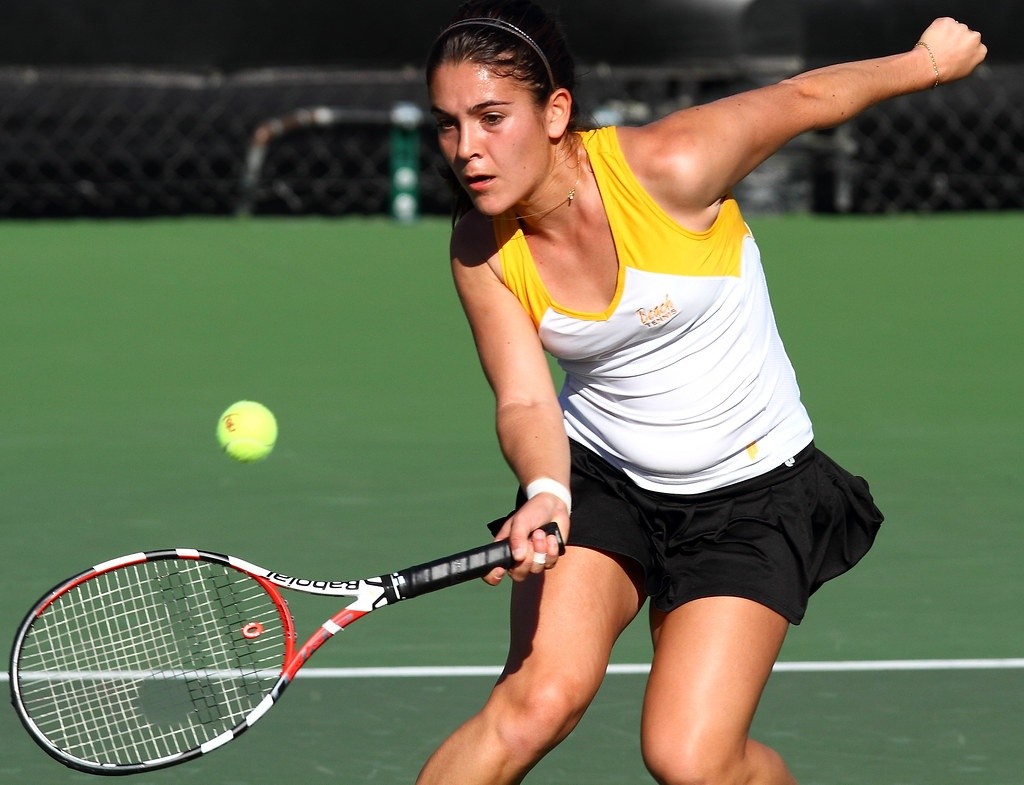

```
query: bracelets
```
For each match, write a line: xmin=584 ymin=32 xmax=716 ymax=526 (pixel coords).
xmin=526 ymin=477 xmax=572 ymax=515
xmin=914 ymin=41 xmax=940 ymax=89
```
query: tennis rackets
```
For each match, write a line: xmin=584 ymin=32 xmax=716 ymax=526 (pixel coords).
xmin=12 ymin=521 xmax=570 ymax=777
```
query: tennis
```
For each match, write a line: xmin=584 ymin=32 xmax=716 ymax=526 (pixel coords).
xmin=216 ymin=401 xmax=279 ymax=462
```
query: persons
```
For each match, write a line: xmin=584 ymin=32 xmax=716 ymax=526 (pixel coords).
xmin=417 ymin=0 xmax=987 ymax=785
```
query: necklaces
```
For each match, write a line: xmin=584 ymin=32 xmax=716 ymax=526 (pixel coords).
xmin=500 ymin=137 xmax=580 ymax=220
